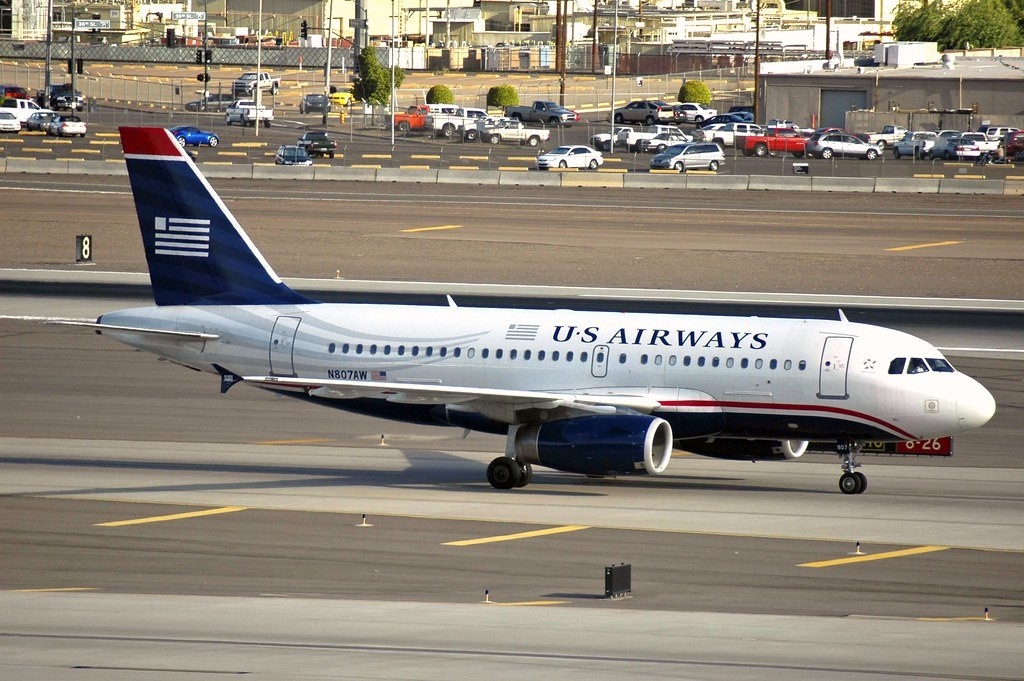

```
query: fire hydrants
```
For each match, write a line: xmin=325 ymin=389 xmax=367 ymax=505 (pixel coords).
xmin=339 ymin=110 xmax=347 ymax=125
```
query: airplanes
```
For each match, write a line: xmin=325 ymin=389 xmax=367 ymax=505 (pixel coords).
xmin=1 ymin=127 xmax=997 ymax=496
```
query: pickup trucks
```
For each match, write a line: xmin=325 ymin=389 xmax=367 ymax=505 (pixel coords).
xmin=295 ymin=132 xmax=338 ymax=159
xmin=732 ymin=128 xmax=809 ymax=159
xmin=892 ymin=131 xmax=938 ymax=160
xmin=504 ymin=101 xmax=579 ymax=129
xmin=230 ymin=72 xmax=282 ymax=96
xmin=386 ymin=105 xmax=551 ymax=148
xmin=224 ymin=100 xmax=275 ymax=128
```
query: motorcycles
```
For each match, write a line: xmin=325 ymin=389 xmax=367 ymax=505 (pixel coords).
xmin=976 ymin=150 xmax=1011 ymax=166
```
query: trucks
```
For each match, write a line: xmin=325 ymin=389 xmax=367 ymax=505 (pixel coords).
xmin=852 ymin=125 xmax=911 ymax=150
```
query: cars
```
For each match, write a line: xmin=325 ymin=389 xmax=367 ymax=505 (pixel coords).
xmin=0 ymin=84 xmax=87 ymax=137
xmin=927 ymin=135 xmax=982 ymax=161
xmin=300 ymin=85 xmax=359 ymax=115
xmin=936 ymin=124 xmax=1024 ymax=162
xmin=588 ymin=97 xmax=849 ymax=156
xmin=184 ymin=94 xmax=239 ymax=112
xmin=535 ymin=145 xmax=604 ymax=171
xmin=168 ymin=126 xmax=220 ymax=147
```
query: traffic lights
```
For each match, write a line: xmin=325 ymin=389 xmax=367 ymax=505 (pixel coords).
xmin=78 ymin=59 xmax=84 ymax=74
xmin=196 ymin=74 xmax=210 ymax=82
xmin=195 ymin=49 xmax=214 ymax=65
xmin=300 ymin=19 xmax=308 ymax=40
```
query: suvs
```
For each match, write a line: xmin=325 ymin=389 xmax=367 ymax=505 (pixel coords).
xmin=804 ymin=133 xmax=884 ymax=161
xmin=275 ymin=145 xmax=314 ymax=167
xmin=648 ymin=142 xmax=726 ymax=172
